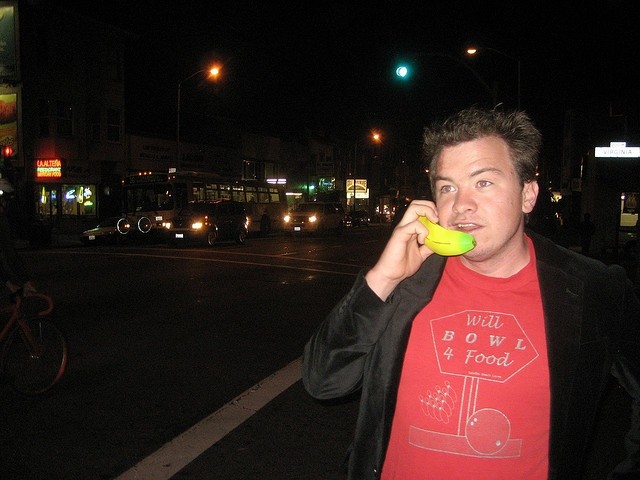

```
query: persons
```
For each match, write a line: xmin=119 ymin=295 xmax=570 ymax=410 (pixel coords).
xmin=302 ymin=102 xmax=639 ymax=480
xmin=576 ymin=212 xmax=596 ymax=256
xmin=0 ymin=180 xmax=37 ymax=299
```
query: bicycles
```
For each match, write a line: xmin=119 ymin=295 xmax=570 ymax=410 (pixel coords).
xmin=117 ymin=207 xmax=154 ymax=235
xmin=0 ymin=276 xmax=69 ymax=402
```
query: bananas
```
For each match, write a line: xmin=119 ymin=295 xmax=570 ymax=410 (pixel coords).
xmin=418 ymin=216 xmax=477 ymax=257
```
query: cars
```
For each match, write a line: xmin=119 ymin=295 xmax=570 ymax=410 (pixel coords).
xmin=344 ymin=211 xmax=371 ymax=230
xmin=80 ymin=216 xmax=120 ymax=242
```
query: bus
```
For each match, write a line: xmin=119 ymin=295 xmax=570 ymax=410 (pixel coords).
xmin=115 ymin=171 xmax=287 ymax=243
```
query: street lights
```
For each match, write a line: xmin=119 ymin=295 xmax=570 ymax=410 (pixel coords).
xmin=353 ymin=131 xmax=380 ymax=211
xmin=177 ymin=64 xmax=222 ymax=172
xmin=394 ymin=52 xmax=488 ymax=91
xmin=465 ymin=45 xmax=523 ymax=113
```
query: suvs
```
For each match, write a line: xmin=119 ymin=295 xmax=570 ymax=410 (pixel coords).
xmin=282 ymin=201 xmax=345 ymax=236
xmin=162 ymin=202 xmax=250 ymax=246
